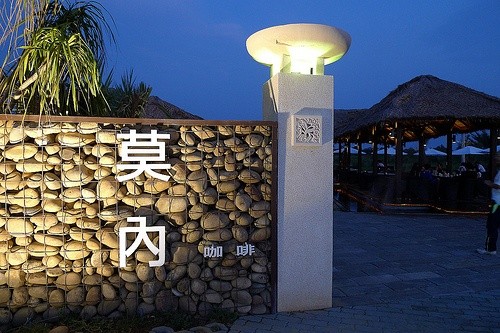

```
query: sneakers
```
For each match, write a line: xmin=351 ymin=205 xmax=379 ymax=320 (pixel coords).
xmin=476 ymin=248 xmax=496 ymax=255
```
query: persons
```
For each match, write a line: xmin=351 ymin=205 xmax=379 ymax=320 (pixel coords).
xmin=410 ymin=163 xmax=428 ymax=178
xmin=437 ymin=164 xmax=446 ymax=175
xmin=475 ymin=150 xmax=500 ymax=255
xmin=459 ymin=159 xmax=477 ymax=173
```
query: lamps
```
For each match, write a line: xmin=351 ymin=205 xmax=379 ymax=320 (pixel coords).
xmin=404 ymin=130 xmax=415 ymax=138
xmin=424 ymin=125 xmax=438 ymax=136
xmin=454 ymin=119 xmax=468 ymax=129
